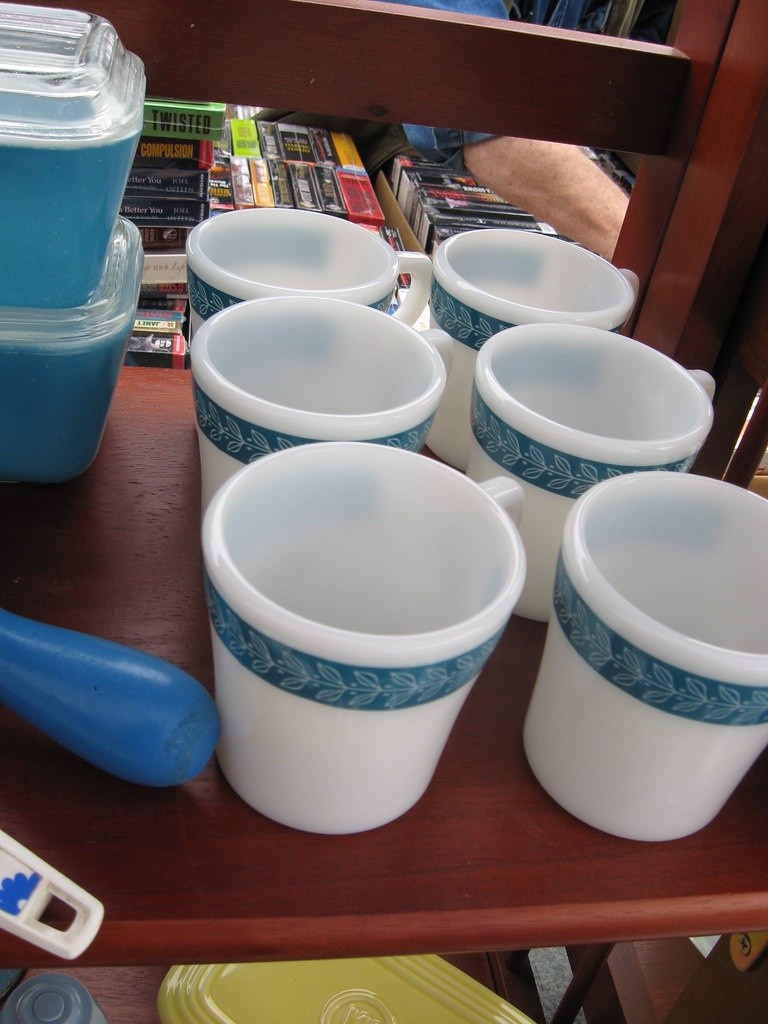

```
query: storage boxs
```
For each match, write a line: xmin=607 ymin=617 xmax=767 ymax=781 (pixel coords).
xmin=1 ymin=3 xmax=147 ymax=311
xmin=372 ymin=162 xmax=435 ymax=281
xmin=0 ymin=217 xmax=144 ymax=483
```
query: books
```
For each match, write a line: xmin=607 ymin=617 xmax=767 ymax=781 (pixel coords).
xmin=119 ymin=98 xmax=578 ymax=368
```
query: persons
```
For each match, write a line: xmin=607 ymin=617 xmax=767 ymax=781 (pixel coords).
xmin=378 ymin=0 xmax=629 ymax=260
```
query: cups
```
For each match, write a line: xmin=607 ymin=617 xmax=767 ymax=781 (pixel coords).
xmin=200 ymin=442 xmax=526 ymax=835
xmin=524 ymin=471 xmax=768 ymax=842
xmin=464 ymin=324 xmax=716 ymax=623
xmin=191 ymin=297 xmax=447 ymax=519
xmin=425 ymin=229 xmax=640 ymax=470
xmin=187 ymin=209 xmax=431 ymax=335
